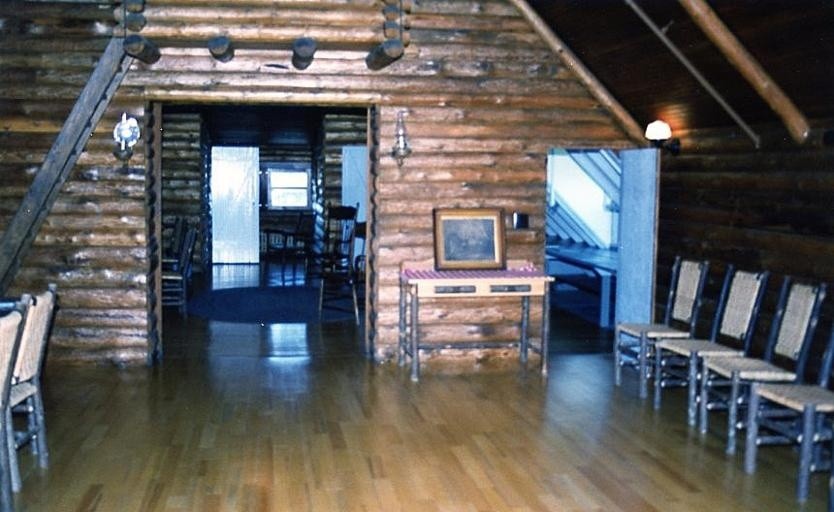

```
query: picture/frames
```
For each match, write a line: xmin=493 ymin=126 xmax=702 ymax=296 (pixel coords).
xmin=432 ymin=207 xmax=506 ymax=270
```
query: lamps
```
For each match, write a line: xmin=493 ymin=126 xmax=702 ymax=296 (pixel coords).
xmin=644 ymin=119 xmax=681 ymax=157
xmin=112 ymin=111 xmax=141 ymax=168
xmin=391 ymin=111 xmax=411 ymax=166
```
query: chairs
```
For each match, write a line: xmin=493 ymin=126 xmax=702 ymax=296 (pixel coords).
xmin=259 ymin=199 xmax=368 ymax=326
xmin=0 ymin=281 xmax=59 ymax=512
xmin=744 ymin=327 xmax=834 ymax=512
xmin=616 ymin=256 xmax=709 ymax=400
xmin=161 ymin=214 xmax=197 ymax=321
xmin=698 ymin=275 xmax=826 ymax=457
xmin=653 ymin=264 xmax=770 ymax=426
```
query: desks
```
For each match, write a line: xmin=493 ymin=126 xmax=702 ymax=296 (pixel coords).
xmin=398 ymin=263 xmax=557 ymax=381
